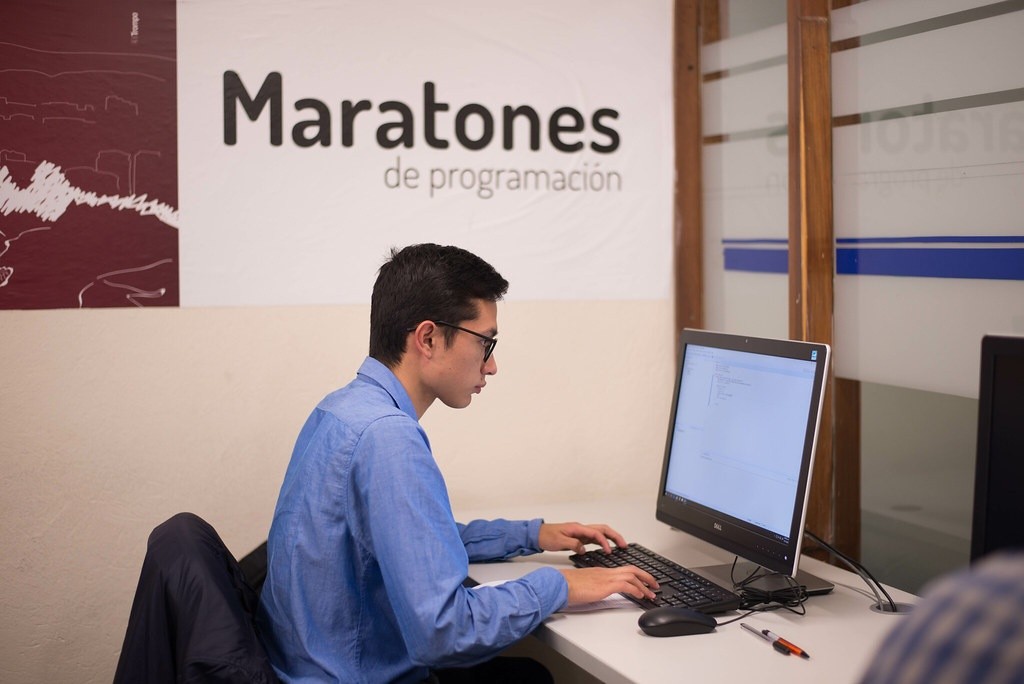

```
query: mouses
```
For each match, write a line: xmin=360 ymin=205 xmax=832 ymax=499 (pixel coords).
xmin=638 ymin=607 xmax=718 ymax=637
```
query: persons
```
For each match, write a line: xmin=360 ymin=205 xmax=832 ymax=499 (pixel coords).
xmin=258 ymin=244 xmax=659 ymax=684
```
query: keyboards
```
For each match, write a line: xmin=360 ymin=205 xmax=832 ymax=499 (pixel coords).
xmin=568 ymin=542 xmax=740 ymax=614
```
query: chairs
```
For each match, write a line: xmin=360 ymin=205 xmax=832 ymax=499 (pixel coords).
xmin=113 ymin=512 xmax=554 ymax=684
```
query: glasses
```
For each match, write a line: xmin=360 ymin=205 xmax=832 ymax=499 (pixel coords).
xmin=407 ymin=320 xmax=497 ymax=363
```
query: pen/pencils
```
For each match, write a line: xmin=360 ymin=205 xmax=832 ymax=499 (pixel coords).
xmin=740 ymin=623 xmax=790 ymax=655
xmin=761 ymin=628 xmax=810 ymax=659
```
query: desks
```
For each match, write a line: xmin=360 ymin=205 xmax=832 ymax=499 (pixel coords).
xmin=455 ymin=503 xmax=925 ymax=684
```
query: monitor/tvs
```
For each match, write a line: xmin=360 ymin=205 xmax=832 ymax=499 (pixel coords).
xmin=970 ymin=335 xmax=1024 ymax=560
xmin=655 ymin=329 xmax=835 ymax=601
